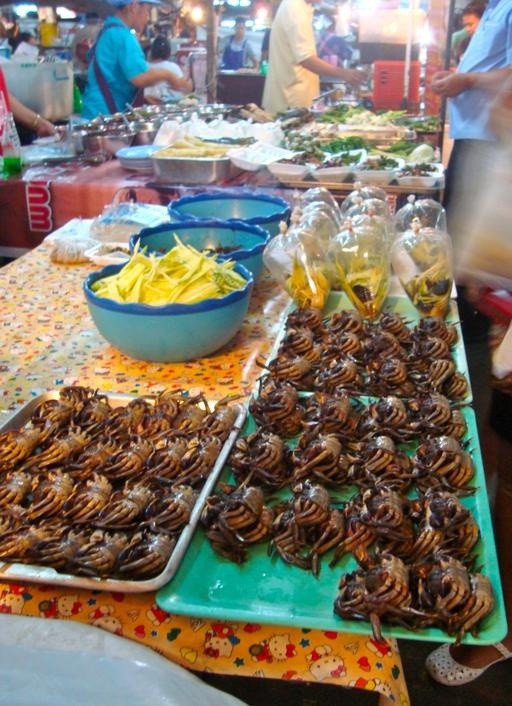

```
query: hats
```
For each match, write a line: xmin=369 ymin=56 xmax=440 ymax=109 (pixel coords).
xmin=107 ymin=0 xmax=163 ymax=8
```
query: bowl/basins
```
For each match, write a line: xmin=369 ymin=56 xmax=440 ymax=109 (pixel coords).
xmin=82 ymin=130 xmax=137 ymax=158
xmin=166 ymin=190 xmax=289 ymax=239
xmin=133 ymin=103 xmax=243 ymax=144
xmin=131 ymin=219 xmax=270 ymax=293
xmin=130 ymin=121 xmax=161 ymax=144
xmin=95 ymin=112 xmax=140 ymax=130
xmin=80 ymin=256 xmax=254 ymax=362
xmin=64 ymin=123 xmax=94 ymax=153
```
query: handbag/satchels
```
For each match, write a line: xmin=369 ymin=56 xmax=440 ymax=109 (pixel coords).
xmin=96 ymin=21 xmax=146 ymax=118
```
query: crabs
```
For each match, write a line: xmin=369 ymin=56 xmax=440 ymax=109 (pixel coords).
xmin=1 ymin=384 xmax=245 ymax=579
xmin=200 ymin=308 xmax=494 ymax=648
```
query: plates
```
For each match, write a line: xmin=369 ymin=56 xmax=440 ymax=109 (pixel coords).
xmin=114 ymin=145 xmax=165 ymax=175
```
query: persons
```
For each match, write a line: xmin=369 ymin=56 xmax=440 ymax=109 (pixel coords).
xmin=80 ymin=0 xmax=181 ymax=120
xmin=312 ymin=13 xmax=353 ymax=68
xmin=428 ymin=0 xmax=512 ymax=237
xmin=462 ymin=2 xmax=486 ymax=36
xmin=144 ymin=36 xmax=184 ymax=105
xmin=0 ymin=65 xmax=60 ymax=157
xmin=71 ymin=12 xmax=102 ymax=69
xmin=150 ymin=24 xmax=165 ymax=44
xmin=422 ymin=74 xmax=512 ymax=685
xmin=261 ymin=0 xmax=366 ymax=115
xmin=261 ymin=27 xmax=271 ymax=64
xmin=14 ymin=31 xmax=37 ymax=56
xmin=0 ymin=15 xmax=15 ymax=59
xmin=172 ymin=14 xmax=196 ymax=47
xmin=217 ymin=16 xmax=258 ymax=73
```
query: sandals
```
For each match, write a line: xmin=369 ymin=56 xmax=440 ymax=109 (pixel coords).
xmin=426 ymin=642 xmax=512 ymax=687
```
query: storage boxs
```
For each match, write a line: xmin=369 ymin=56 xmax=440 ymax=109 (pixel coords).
xmin=3 ymin=61 xmax=74 ymax=121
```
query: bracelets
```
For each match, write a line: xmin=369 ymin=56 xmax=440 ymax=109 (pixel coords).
xmin=32 ymin=113 xmax=40 ymax=128
